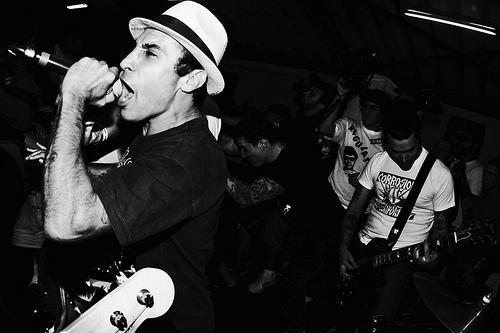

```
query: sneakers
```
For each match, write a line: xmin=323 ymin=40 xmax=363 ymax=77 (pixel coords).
xmin=249 ymin=268 xmax=277 ymax=294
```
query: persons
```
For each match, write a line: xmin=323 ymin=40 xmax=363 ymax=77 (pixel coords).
xmin=24 ymin=0 xmax=228 ymax=333
xmin=0 ymin=39 xmax=500 ymax=333
xmin=325 ymin=104 xmax=456 ymax=333
xmin=197 ymin=114 xmax=347 ymax=293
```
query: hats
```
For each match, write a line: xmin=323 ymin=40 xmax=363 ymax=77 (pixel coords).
xmin=129 ymin=2 xmax=228 ymax=95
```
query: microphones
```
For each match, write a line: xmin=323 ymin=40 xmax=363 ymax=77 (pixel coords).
xmin=22 ymin=46 xmax=123 ymax=97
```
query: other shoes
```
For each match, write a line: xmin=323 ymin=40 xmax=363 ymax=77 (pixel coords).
xmin=304 ymin=289 xmax=318 ymax=302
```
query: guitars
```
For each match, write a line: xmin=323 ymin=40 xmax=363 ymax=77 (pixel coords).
xmin=336 ymin=217 xmax=498 ymax=308
xmin=45 ymin=259 xmax=175 ymax=333
